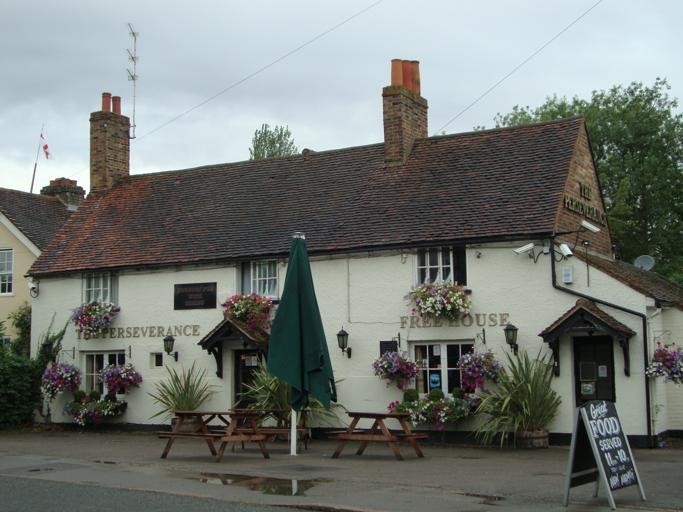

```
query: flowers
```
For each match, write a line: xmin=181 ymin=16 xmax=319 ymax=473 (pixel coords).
xmin=645 ymin=337 xmax=683 ymax=386
xmin=69 ymin=299 xmax=120 ymax=335
xmin=42 ymin=361 xmax=142 ymax=429
xmin=372 ymin=348 xmax=504 ymax=432
xmin=220 ymin=292 xmax=274 ymax=336
xmin=401 ymin=279 xmax=471 ymax=322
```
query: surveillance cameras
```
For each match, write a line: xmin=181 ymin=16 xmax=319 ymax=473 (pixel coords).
xmin=559 ymin=241 xmax=575 ymax=259
xmin=581 ymin=217 xmax=603 ymax=235
xmin=27 ymin=281 xmax=36 ymax=291
xmin=514 ymin=241 xmax=536 ymax=258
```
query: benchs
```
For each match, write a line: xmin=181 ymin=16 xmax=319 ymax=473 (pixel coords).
xmin=153 ymin=427 xmax=310 ymax=464
xmin=326 ymin=428 xmax=429 ymax=461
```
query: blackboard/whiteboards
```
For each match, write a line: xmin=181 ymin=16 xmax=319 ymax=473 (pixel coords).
xmin=582 ymin=399 xmax=639 ymax=490
xmin=564 ymin=407 xmax=601 ymax=488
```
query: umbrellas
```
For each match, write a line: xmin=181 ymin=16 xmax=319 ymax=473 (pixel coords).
xmin=266 ymin=229 xmax=337 ymax=456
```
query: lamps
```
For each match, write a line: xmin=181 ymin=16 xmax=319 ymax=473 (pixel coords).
xmin=504 ymin=323 xmax=519 ymax=354
xmin=337 ymin=325 xmax=351 ymax=358
xmin=164 ymin=334 xmax=179 ymax=363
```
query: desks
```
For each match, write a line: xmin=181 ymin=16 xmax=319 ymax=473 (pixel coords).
xmin=331 ymin=410 xmax=424 ymax=461
xmin=160 ymin=408 xmax=289 ymax=462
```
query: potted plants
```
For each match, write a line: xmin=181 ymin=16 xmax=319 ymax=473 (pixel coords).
xmin=466 ymin=348 xmax=564 ymax=450
xmin=237 ymin=354 xmax=350 ymax=442
xmin=145 ymin=360 xmax=223 ymax=433
